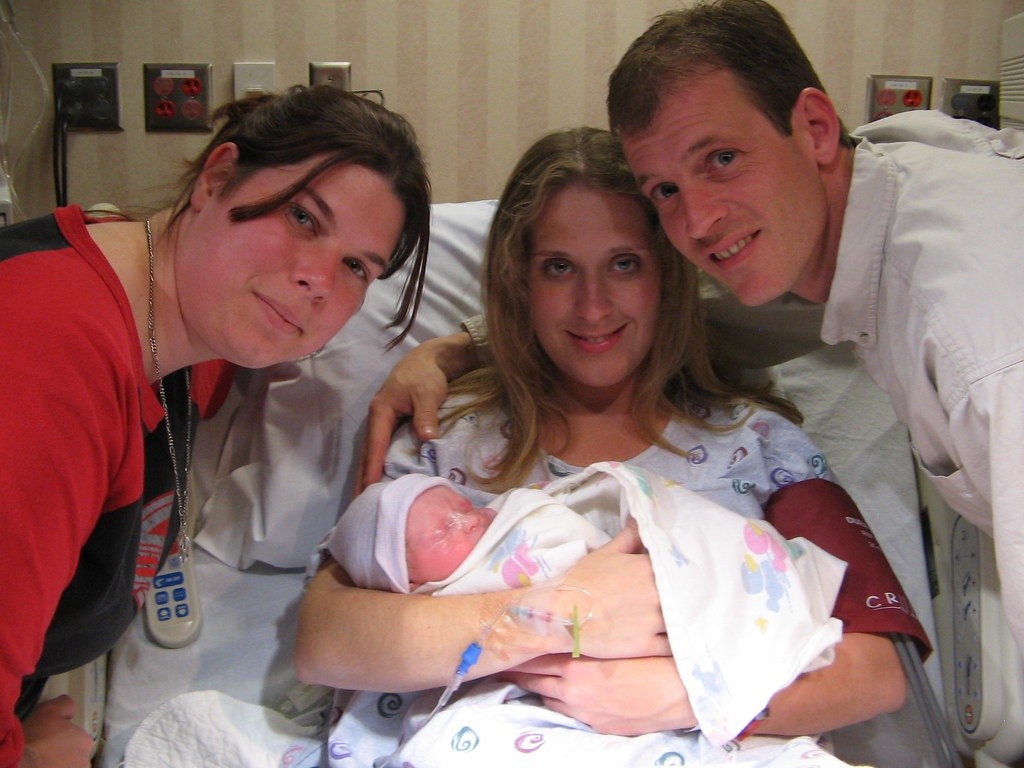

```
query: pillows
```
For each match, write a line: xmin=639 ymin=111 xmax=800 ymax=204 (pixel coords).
xmin=190 ymin=199 xmax=792 ymax=572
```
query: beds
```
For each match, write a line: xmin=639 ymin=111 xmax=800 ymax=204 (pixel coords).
xmin=38 ymin=266 xmax=1024 ymax=768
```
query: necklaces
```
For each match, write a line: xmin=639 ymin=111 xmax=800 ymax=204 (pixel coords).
xmin=143 ymin=221 xmax=192 ymax=565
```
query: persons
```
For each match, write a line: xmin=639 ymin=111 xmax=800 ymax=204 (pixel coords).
xmin=292 ymin=128 xmax=934 ymax=735
xmin=352 ymin=0 xmax=1021 ymax=768
xmin=313 ymin=460 xmax=851 ymax=768
xmin=0 ymin=84 xmax=431 ymax=767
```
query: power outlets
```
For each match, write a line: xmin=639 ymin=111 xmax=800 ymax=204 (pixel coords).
xmin=942 ymin=78 xmax=1000 ymax=131
xmin=309 ymin=60 xmax=352 ymax=93
xmin=52 ymin=60 xmax=125 ymax=133
xmin=867 ymin=72 xmax=933 ymax=122
xmin=143 ymin=62 xmax=214 ymax=133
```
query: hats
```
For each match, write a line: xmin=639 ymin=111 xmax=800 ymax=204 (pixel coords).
xmin=302 ymin=473 xmax=468 ymax=594
